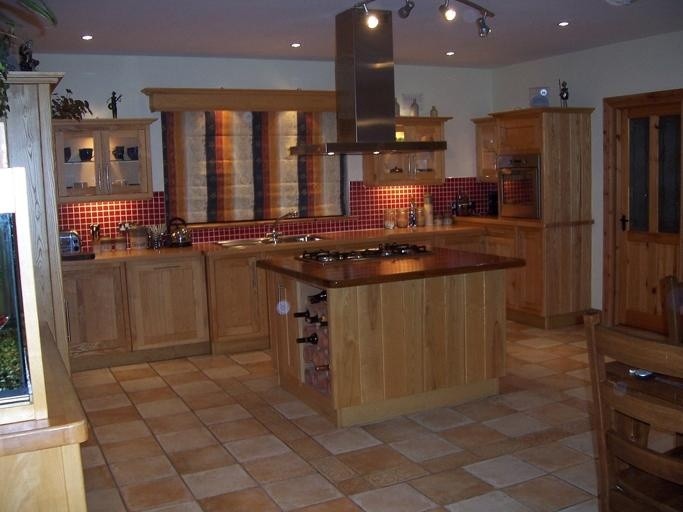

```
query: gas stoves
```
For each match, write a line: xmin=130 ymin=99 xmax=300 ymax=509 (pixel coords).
xmin=295 ymin=241 xmax=430 ymax=266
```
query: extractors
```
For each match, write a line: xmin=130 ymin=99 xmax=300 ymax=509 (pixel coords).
xmin=287 ymin=6 xmax=448 ymax=158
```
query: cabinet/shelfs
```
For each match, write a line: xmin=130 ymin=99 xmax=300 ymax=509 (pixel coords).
xmin=61 ymin=253 xmax=208 ymax=373
xmin=363 ymin=115 xmax=451 ymax=187
xmin=264 ymin=269 xmax=332 ymax=407
xmin=200 ymin=241 xmax=271 ymax=358
xmin=2 ymin=66 xmax=73 ymax=379
xmin=479 ymin=220 xmax=596 ymax=330
xmin=475 ymin=109 xmax=595 ymax=220
xmin=51 ymin=116 xmax=160 ymax=200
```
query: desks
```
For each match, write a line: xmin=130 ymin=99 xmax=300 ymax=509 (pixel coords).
xmin=0 ymin=323 xmax=91 ymax=512
xmin=602 ymin=355 xmax=682 ymax=462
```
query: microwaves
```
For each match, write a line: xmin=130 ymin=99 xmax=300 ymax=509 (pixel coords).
xmin=59 ymin=231 xmax=80 ymax=253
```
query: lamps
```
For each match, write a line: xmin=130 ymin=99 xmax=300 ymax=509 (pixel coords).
xmin=356 ymin=0 xmax=493 ymax=38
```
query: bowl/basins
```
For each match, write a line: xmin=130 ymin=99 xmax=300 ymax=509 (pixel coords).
xmin=64 ymin=146 xmax=71 ymax=163
xmin=126 ymin=146 xmax=139 ymax=161
xmin=78 ymin=148 xmax=94 ymax=161
xmin=112 ymin=146 xmax=124 ymax=160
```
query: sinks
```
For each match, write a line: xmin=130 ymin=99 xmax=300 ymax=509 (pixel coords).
xmin=217 ymin=233 xmax=331 ymax=246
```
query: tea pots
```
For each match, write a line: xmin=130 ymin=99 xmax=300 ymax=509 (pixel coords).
xmin=164 ymin=218 xmax=192 ymax=247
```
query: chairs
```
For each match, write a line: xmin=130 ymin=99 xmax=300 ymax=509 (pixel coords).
xmin=584 ymin=309 xmax=682 ymax=512
xmin=658 ymin=276 xmax=681 ymax=343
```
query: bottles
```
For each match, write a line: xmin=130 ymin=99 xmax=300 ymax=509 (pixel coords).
xmin=415 ymin=201 xmax=425 ymax=226
xmin=442 ymin=213 xmax=452 ymax=225
xmin=433 ymin=215 xmax=441 ymax=225
xmin=383 ymin=208 xmax=394 ymax=229
xmin=430 ymin=105 xmax=438 ymax=118
xmin=394 ymin=97 xmax=401 ymax=118
xmin=294 ymin=332 xmax=318 ymax=346
xmin=394 ymin=208 xmax=408 ymax=227
xmin=314 ymin=364 xmax=329 ymax=372
xmin=408 ymin=97 xmax=420 ymax=117
xmin=100 ymin=224 xmax=162 ymax=251
xmin=291 ymin=310 xmax=328 ymax=330
xmin=422 ymin=193 xmax=433 ymax=226
xmin=309 ymin=291 xmax=327 ymax=304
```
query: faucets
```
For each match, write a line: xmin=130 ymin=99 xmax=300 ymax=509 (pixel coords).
xmin=268 ymin=210 xmax=298 ymax=245
